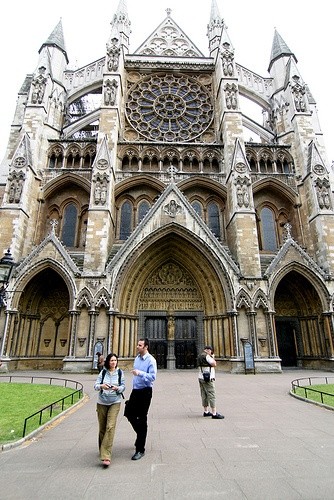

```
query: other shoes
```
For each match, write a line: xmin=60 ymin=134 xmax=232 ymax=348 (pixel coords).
xmin=203 ymin=412 xmax=212 ymax=417
xmin=102 ymin=460 xmax=110 ymax=465
xmin=212 ymin=412 xmax=224 ymax=419
xmin=132 ymin=452 xmax=145 ymax=460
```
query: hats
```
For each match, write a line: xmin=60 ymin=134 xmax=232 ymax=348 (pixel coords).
xmin=205 ymin=346 xmax=213 ymax=350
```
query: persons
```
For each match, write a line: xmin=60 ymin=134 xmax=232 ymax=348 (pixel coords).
xmin=121 ymin=337 xmax=157 ymax=461
xmin=96 ymin=352 xmax=104 ymax=374
xmin=196 ymin=346 xmax=225 ymax=419
xmin=93 ymin=353 xmax=125 ymax=467
